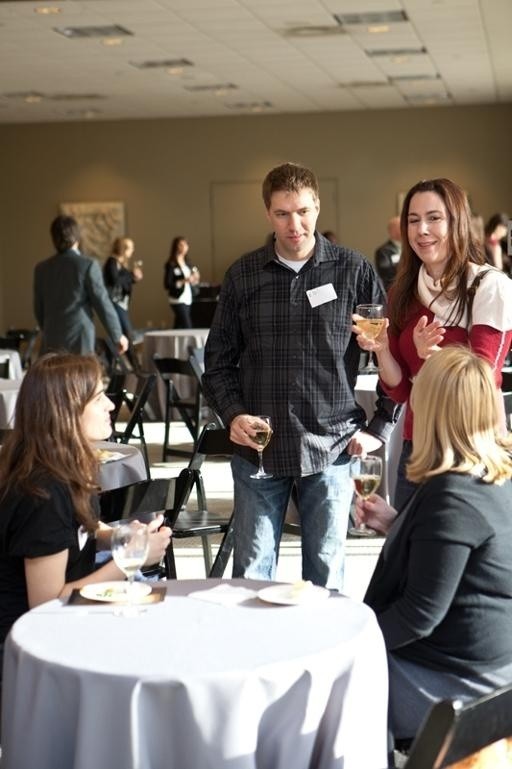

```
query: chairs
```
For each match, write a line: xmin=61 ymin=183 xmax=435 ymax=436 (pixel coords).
xmin=390 ymin=683 xmax=512 ymax=769
xmin=1 ymin=329 xmax=379 ymax=579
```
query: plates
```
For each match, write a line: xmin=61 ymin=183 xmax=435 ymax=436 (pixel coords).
xmin=256 ymin=583 xmax=332 ymax=607
xmin=75 ymin=581 xmax=154 ymax=605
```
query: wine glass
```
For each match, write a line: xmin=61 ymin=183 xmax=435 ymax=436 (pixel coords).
xmin=355 ymin=302 xmax=385 ymax=375
xmin=348 ymin=453 xmax=383 ymax=539
xmin=247 ymin=414 xmax=274 ymax=481
xmin=110 ymin=522 xmax=151 ymax=617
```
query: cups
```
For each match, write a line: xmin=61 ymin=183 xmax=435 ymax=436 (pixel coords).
xmin=133 ymin=260 xmax=143 ymax=271
xmin=190 ymin=264 xmax=200 ymax=278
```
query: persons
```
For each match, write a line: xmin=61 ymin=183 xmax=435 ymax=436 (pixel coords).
xmin=33 ymin=216 xmax=130 ymax=360
xmin=362 ymin=345 xmax=512 ymax=769
xmin=0 ymin=353 xmax=173 ymax=687
xmin=102 ymin=237 xmax=143 ymax=338
xmin=163 ymin=237 xmax=200 ymax=329
xmin=351 ymin=177 xmax=512 ymax=512
xmin=201 ymin=162 xmax=407 ymax=590
xmin=374 ymin=214 xmax=508 ymax=294
